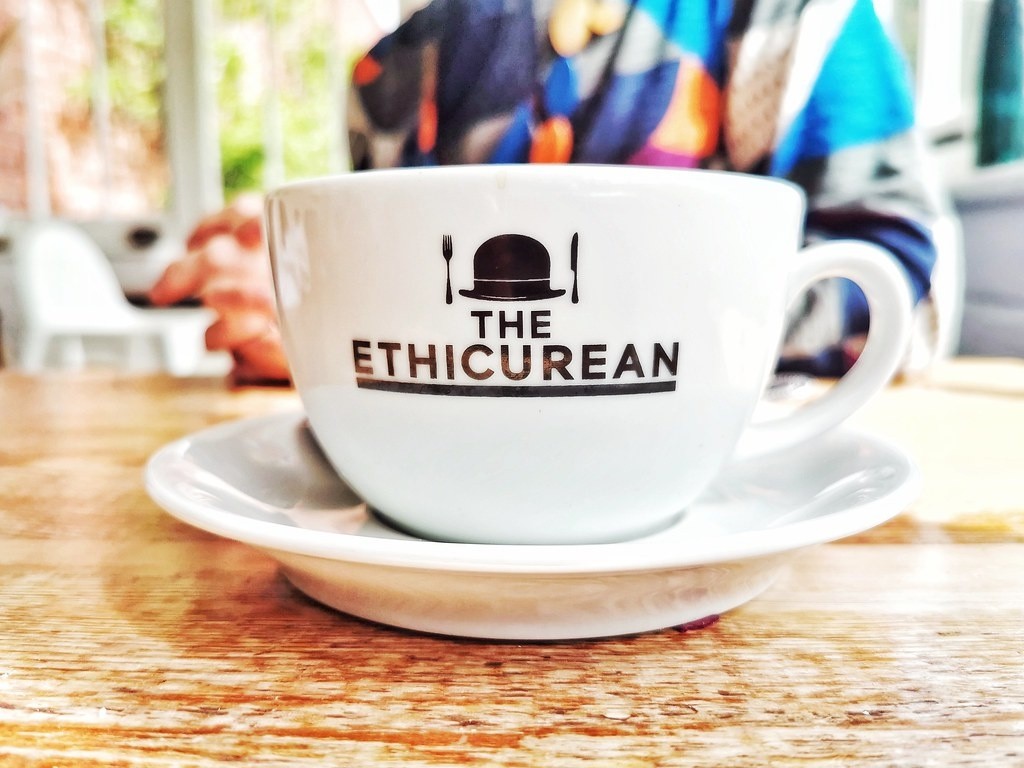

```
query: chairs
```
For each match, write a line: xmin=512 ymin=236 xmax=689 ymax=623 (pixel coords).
xmin=15 ymin=227 xmax=233 ymax=375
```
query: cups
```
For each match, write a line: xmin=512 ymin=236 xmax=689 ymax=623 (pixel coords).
xmin=264 ymin=164 xmax=912 ymax=545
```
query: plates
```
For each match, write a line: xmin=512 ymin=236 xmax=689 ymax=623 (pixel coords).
xmin=143 ymin=387 xmax=919 ymax=641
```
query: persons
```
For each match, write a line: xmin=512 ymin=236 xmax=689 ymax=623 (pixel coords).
xmin=148 ymin=0 xmax=962 ymax=385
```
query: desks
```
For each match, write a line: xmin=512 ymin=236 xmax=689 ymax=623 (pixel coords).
xmin=0 ymin=359 xmax=1024 ymax=766
